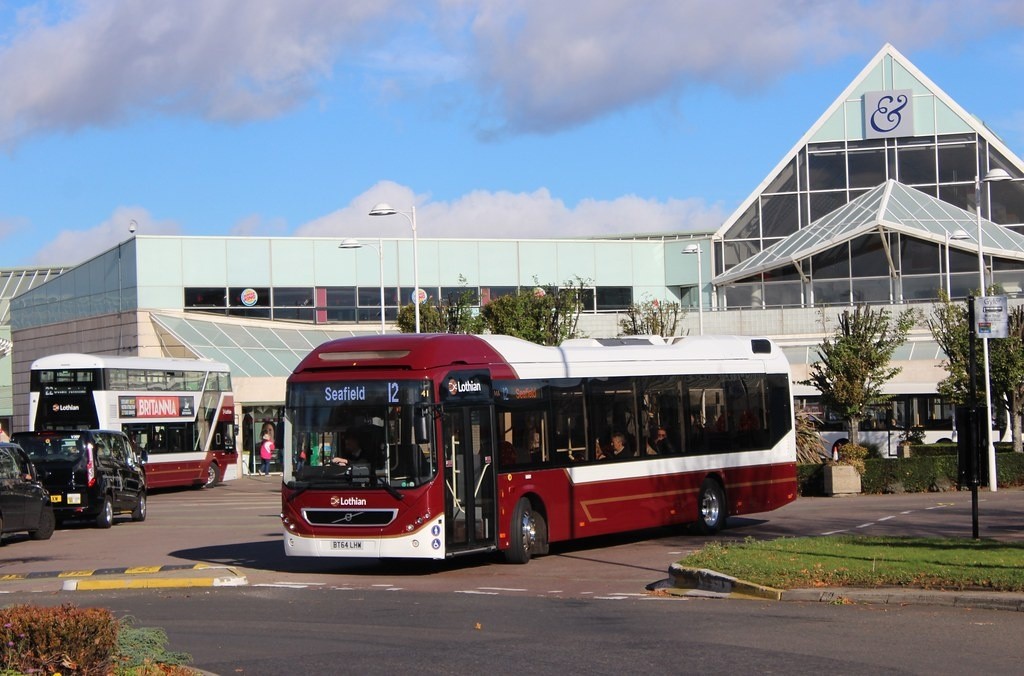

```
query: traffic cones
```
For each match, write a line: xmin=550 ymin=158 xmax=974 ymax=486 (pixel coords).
xmin=833 ymin=446 xmax=839 ymax=462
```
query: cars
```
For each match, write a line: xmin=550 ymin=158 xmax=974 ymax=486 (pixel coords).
xmin=0 ymin=441 xmax=56 ymax=540
xmin=9 ymin=429 xmax=149 ymax=528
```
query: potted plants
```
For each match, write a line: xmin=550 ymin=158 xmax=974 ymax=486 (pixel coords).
xmin=824 ymin=443 xmax=868 ymax=495
xmin=897 ymin=439 xmax=912 ymax=458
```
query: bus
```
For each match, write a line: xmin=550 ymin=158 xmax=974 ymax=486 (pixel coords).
xmin=279 ymin=335 xmax=799 ymax=569
xmin=28 ymin=353 xmax=238 ymax=491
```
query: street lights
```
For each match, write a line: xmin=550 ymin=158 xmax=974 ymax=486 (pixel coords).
xmin=367 ymin=196 xmax=421 ymax=336
xmin=335 ymin=236 xmax=387 ymax=337
xmin=951 ymin=230 xmax=998 ymax=493
xmin=681 ymin=243 xmax=704 ymax=336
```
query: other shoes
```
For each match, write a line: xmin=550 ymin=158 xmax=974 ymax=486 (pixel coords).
xmin=265 ymin=474 xmax=271 ymax=477
xmin=258 ymin=470 xmax=262 ymax=476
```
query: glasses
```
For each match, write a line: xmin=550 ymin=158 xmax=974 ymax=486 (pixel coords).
xmin=657 ymin=434 xmax=666 ymax=437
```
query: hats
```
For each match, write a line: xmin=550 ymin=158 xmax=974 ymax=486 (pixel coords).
xmin=263 ymin=434 xmax=271 ymax=440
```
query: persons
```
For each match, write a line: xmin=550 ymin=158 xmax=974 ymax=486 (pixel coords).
xmin=257 ymin=422 xmax=279 ymax=451
xmin=258 ymin=434 xmax=275 ymax=476
xmin=500 ymin=411 xmax=696 ymax=466
xmin=332 ymin=435 xmax=370 ymax=467
xmin=23 ymin=473 xmax=32 ymax=481
xmin=891 ymin=419 xmax=904 ymax=429
